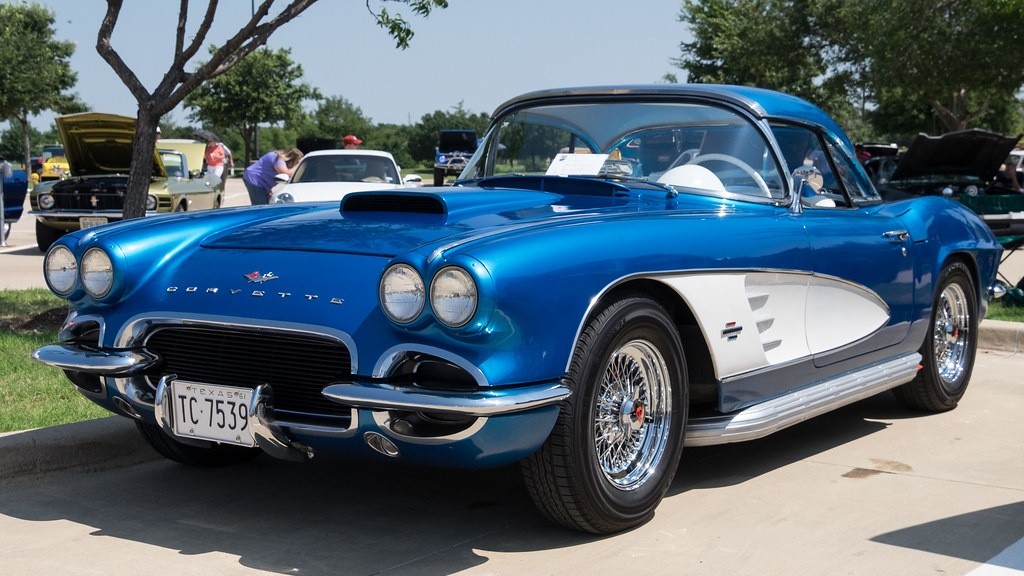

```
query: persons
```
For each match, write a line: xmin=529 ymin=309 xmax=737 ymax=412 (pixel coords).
xmin=156 ymin=126 xmax=161 ymax=139
xmin=202 ymin=139 xmax=234 ymax=194
xmin=242 ymin=147 xmax=304 ymax=206
xmin=994 ymin=163 xmax=1024 ymax=192
xmin=341 ymin=136 xmax=363 ymax=150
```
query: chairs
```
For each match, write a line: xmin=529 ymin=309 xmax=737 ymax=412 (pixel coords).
xmin=311 ymin=161 xmax=337 ymax=182
xmin=360 ymin=161 xmax=385 ymax=182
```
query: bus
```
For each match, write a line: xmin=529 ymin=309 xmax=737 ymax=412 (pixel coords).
xmin=31 ymin=157 xmax=71 ymax=185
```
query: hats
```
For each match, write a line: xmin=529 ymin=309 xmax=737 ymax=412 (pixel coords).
xmin=344 ymin=135 xmax=362 ymax=145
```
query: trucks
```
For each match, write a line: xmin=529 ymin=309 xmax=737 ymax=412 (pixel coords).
xmin=433 ymin=127 xmax=506 ymax=186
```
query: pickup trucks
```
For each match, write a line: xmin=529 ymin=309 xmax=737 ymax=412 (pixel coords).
xmin=853 ymin=128 xmax=1024 ymax=214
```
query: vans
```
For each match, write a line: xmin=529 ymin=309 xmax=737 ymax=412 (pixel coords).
xmin=977 ymin=211 xmax=1024 ymax=308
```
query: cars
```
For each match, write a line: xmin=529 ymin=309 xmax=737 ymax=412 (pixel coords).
xmin=27 ymin=110 xmax=168 ymax=254
xmin=144 ymin=138 xmax=228 ymax=215
xmin=268 ymin=149 xmax=425 ymax=211
xmin=34 ymin=87 xmax=1001 ymax=535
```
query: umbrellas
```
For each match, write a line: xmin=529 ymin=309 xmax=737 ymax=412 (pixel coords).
xmin=194 ymin=130 xmax=220 ymax=142
